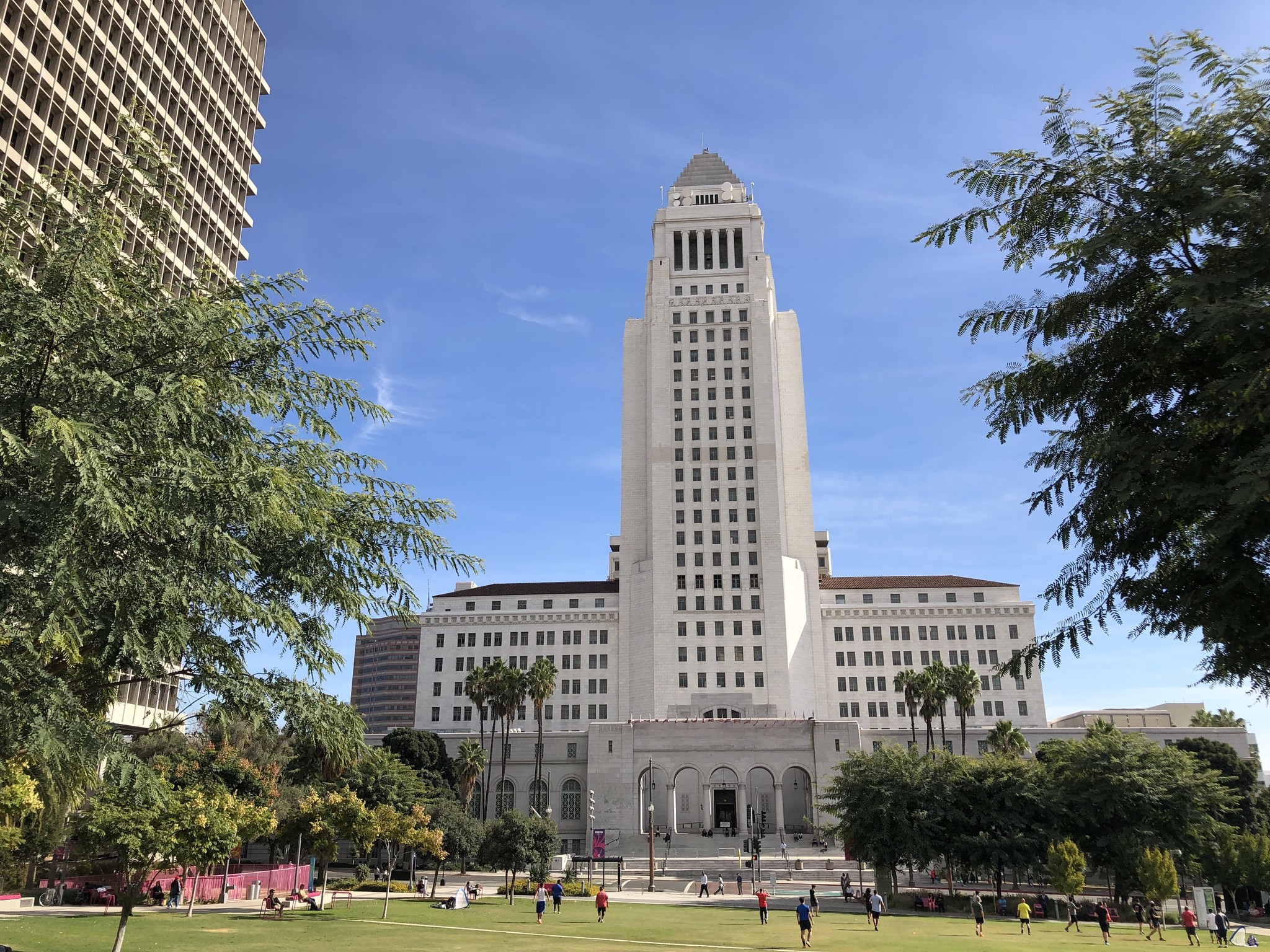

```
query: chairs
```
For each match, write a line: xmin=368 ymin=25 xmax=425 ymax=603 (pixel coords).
xmin=292 ymin=893 xmax=310 ymax=911
xmin=462 ymin=886 xmax=475 ymax=901
xmin=477 ymin=887 xmax=483 ymax=900
xmin=414 ymin=884 xmax=426 ymax=898
xmin=1032 ymin=903 xmax=1045 ymax=919
xmin=89 ymin=888 xmax=115 ymax=906
xmin=147 ymin=889 xmax=162 ymax=905
xmin=259 ymin=898 xmax=281 ymax=920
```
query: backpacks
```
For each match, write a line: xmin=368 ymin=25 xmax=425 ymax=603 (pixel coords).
xmin=439 ymin=897 xmax=456 ymax=909
xmin=475 ymin=884 xmax=480 ymax=888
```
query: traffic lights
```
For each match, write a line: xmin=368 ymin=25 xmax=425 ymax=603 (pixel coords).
xmin=751 ymin=836 xmax=755 ymax=849
xmin=757 ymin=839 xmax=762 ymax=852
xmin=751 ymin=855 xmax=757 ymax=861
xmin=761 ymin=827 xmax=766 ymax=838
xmin=742 ymin=838 xmax=748 ymax=852
xmin=761 ymin=810 xmax=766 ymax=821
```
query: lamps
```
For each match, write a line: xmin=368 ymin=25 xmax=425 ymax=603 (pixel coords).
xmin=794 ymin=858 xmax=804 ymax=870
xmin=652 ymin=768 xmax=656 ymax=790
xmin=793 ymin=768 xmax=798 ymax=790
xmin=825 ymin=858 xmax=834 ymax=870
xmin=722 ymin=768 xmax=727 ymax=791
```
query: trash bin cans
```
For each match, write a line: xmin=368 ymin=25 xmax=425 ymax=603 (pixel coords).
xmin=245 ymin=884 xmax=255 ymax=900
xmin=252 ymin=879 xmax=261 ymax=899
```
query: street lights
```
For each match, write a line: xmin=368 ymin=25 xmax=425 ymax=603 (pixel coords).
xmin=588 ymin=788 xmax=595 ymax=889
xmin=647 ymin=799 xmax=656 ymax=891
xmin=545 ymin=804 xmax=551 ymax=822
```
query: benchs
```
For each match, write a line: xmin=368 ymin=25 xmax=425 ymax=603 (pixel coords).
xmin=915 ymin=897 xmax=939 ymax=911
xmin=536 ymin=883 xmax=564 ymax=897
xmin=331 ymin=891 xmax=352 ymax=910
xmin=849 ymin=890 xmax=861 ymax=904
xmin=1087 ymin=907 xmax=1121 ymax=922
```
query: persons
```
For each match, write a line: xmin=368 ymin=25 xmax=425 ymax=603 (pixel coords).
xmin=165 ymin=876 xmax=182 ymax=909
xmin=466 ymin=881 xmax=479 ymax=900
xmin=595 ymin=886 xmax=609 ymax=923
xmin=372 ymin=864 xmax=388 ymax=881
xmin=998 ymin=895 xmax=1007 ymax=916
xmin=418 ymin=881 xmax=429 ymax=898
xmin=863 ymin=888 xmax=872 ymax=924
xmin=549 ymin=880 xmax=566 ymax=914
xmin=298 ymin=884 xmax=322 ymax=910
xmin=176 ymin=875 xmax=186 ymax=906
xmin=838 ymin=862 xmax=996 ymax=919
xmin=697 ymin=871 xmax=709 ymax=898
xmin=268 ymin=889 xmax=285 ymax=918
xmin=755 ymin=888 xmax=771 ymax=924
xmin=532 ymin=883 xmax=550 ymax=924
xmin=713 ymin=874 xmax=725 ymax=895
xmin=1146 ymin=891 xmax=1231 ymax=948
xmin=869 ymin=890 xmax=886 ymax=931
xmin=1094 ymin=901 xmax=1110 ymax=945
xmin=1039 ymin=890 xmax=1049 ymax=918
xmin=647 ymin=818 xmax=827 ymax=859
xmin=152 ymin=880 xmax=164 ymax=906
xmin=796 ymin=897 xmax=814 ymax=948
xmin=1102 ymin=902 xmax=1114 ymax=938
xmin=1134 ymin=898 xmax=1145 ymax=935
xmin=809 ymin=884 xmax=821 ymax=917
xmin=1015 ymin=898 xmax=1032 ymax=935
xmin=971 ymin=897 xmax=985 ymax=936
xmin=736 ymin=873 xmax=743 ymax=895
xmin=1064 ymin=898 xmax=1082 ymax=933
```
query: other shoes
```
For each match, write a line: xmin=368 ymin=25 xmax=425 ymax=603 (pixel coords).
xmin=310 ymin=908 xmax=315 ymax=910
xmin=761 ymin=923 xmax=764 ymax=925
xmin=427 ymin=894 xmax=429 ymax=896
xmin=159 ymin=903 xmax=163 ymax=906
xmin=176 ymin=906 xmax=181 ymax=909
xmin=803 ymin=945 xmax=807 ymax=948
xmin=315 ymin=909 xmax=322 ymax=911
xmin=477 ymin=895 xmax=479 ymax=896
xmin=171 ymin=902 xmax=173 ymax=908
xmin=423 ymin=897 xmax=425 ymax=898
xmin=280 ymin=915 xmax=284 ymax=917
xmin=806 ymin=941 xmax=811 ymax=947
xmin=475 ymin=899 xmax=478 ymax=900
xmin=166 ymin=906 xmax=170 ymax=909
xmin=764 ymin=918 xmax=767 ymax=924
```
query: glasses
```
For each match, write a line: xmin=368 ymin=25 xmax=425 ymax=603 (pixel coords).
xmin=273 ymin=892 xmax=275 ymax=893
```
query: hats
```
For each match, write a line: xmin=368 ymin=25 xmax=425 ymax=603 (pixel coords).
xmin=175 ymin=876 xmax=181 ymax=879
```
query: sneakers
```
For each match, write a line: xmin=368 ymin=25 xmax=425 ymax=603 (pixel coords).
xmin=537 ymin=919 xmax=540 ymax=923
xmin=598 ymin=917 xmax=601 ymax=922
xmin=539 ymin=921 xmax=542 ymax=924
xmin=554 ymin=911 xmax=557 ymax=913
xmin=558 ymin=912 xmax=561 ymax=914
xmin=601 ymin=921 xmax=604 ymax=923
